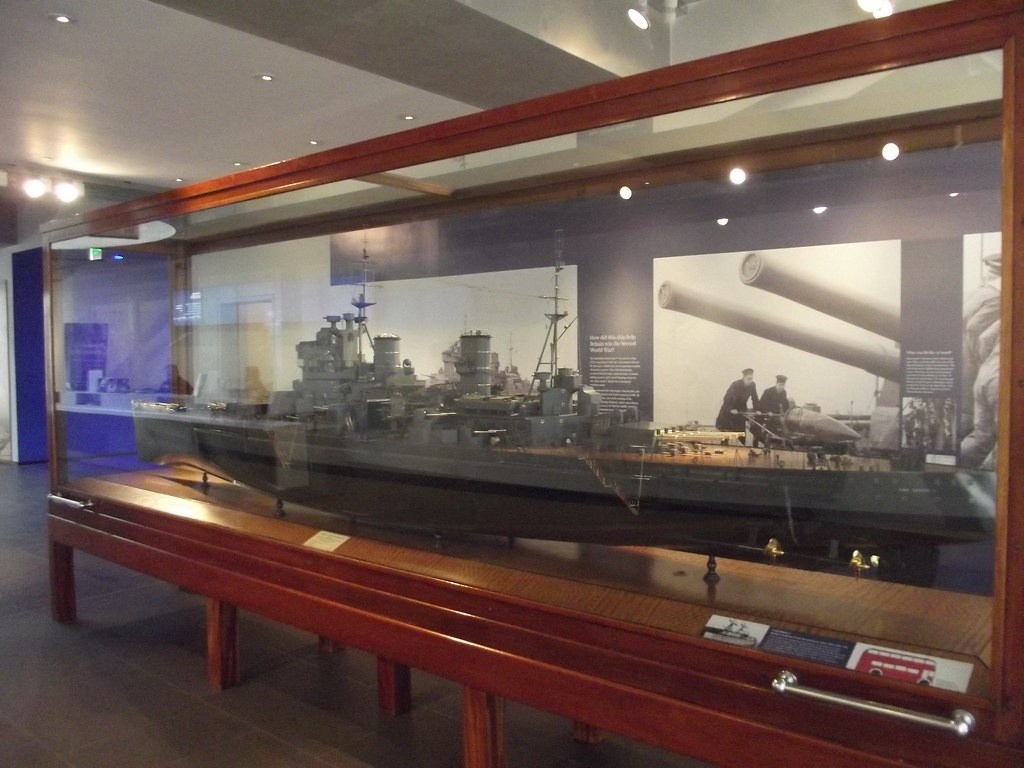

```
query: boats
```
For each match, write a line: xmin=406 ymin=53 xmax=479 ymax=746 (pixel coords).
xmin=129 ymin=245 xmax=1001 ymax=556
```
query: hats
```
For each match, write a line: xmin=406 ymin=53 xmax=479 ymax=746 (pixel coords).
xmin=742 ymin=369 xmax=753 ymax=375
xmin=776 ymin=375 xmax=788 ymax=383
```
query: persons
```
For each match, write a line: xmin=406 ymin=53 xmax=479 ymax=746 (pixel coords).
xmin=160 ymin=365 xmax=194 ymax=394
xmin=228 ymin=365 xmax=268 ymax=403
xmin=903 ymin=397 xmax=952 ymax=451
xmin=715 ymin=368 xmax=789 ymax=447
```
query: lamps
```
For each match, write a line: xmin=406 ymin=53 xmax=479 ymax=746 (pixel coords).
xmin=627 ymin=7 xmax=651 ymax=30
